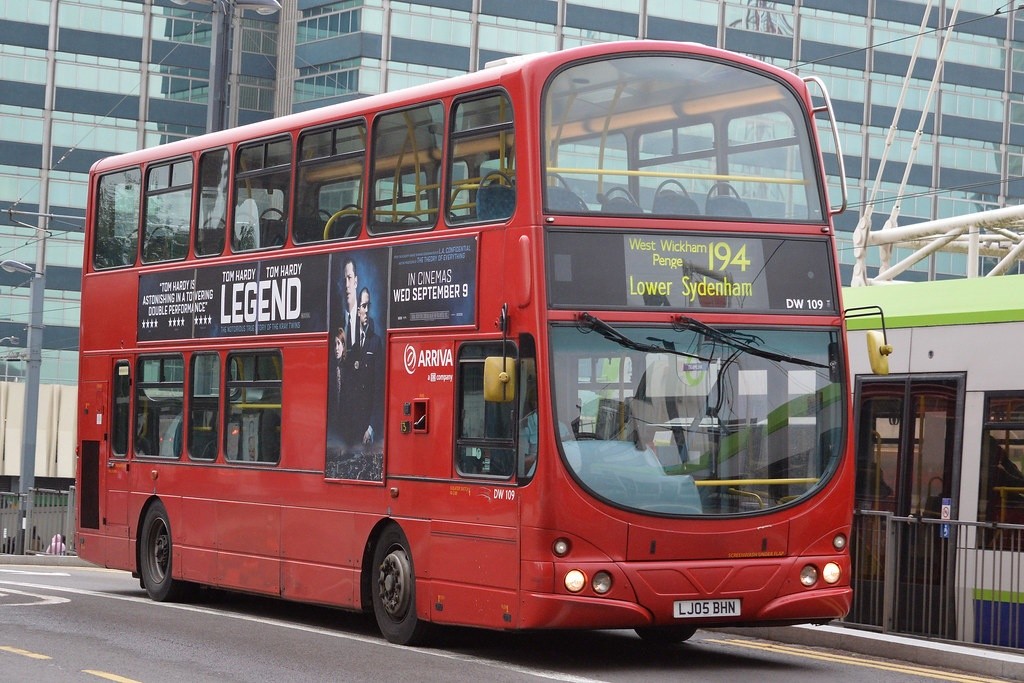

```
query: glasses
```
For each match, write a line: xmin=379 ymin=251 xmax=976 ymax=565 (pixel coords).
xmin=359 ymin=302 xmax=372 ymax=308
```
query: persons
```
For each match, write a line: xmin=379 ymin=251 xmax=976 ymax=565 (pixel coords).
xmin=45 ymin=533 xmax=66 ymax=556
xmin=329 ymin=255 xmax=385 ymax=448
xmin=518 ymin=380 xmax=577 ymax=475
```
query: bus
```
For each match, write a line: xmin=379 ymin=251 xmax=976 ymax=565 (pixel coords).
xmin=602 ymin=275 xmax=1023 ymax=655
xmin=74 ymin=40 xmax=892 ymax=646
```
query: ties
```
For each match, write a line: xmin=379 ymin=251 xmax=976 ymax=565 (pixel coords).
xmin=346 ymin=315 xmax=351 ymax=351
xmin=361 ymin=333 xmax=364 ymax=347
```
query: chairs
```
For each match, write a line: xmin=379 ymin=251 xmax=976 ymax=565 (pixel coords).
xmin=124 ymin=204 xmax=421 ymax=265
xmin=476 ymin=168 xmax=752 ymax=222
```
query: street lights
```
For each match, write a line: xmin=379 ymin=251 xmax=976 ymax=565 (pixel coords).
xmin=0 ymin=260 xmax=46 ymax=556
